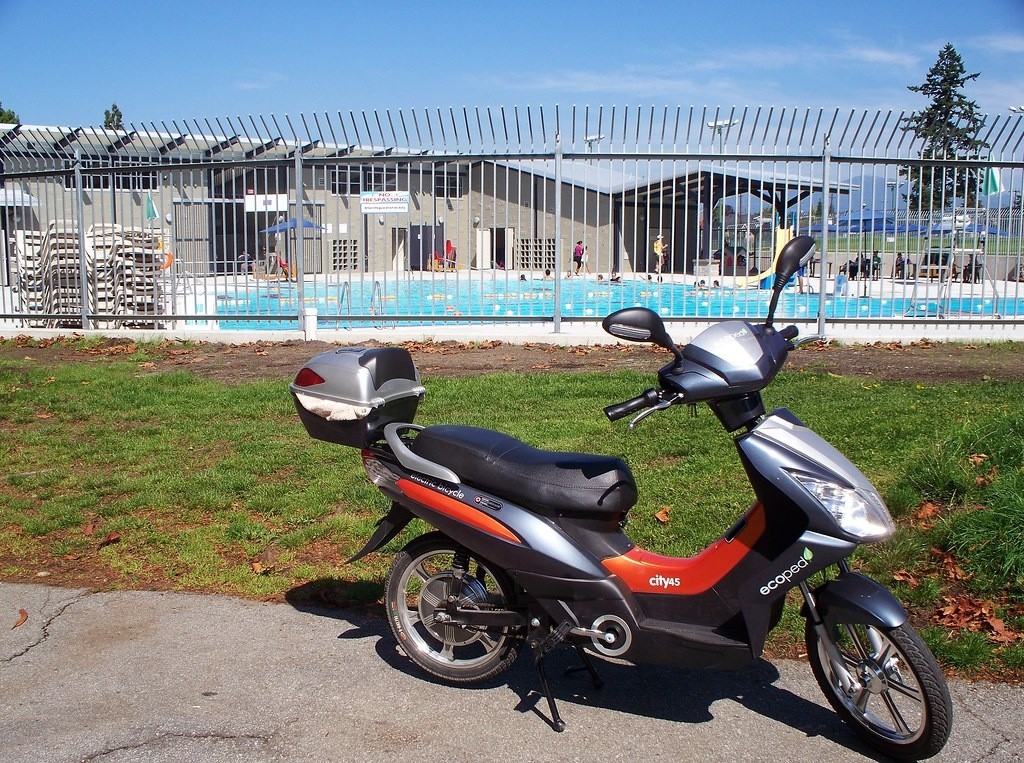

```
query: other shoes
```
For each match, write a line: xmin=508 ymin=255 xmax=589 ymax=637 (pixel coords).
xmin=655 ymin=269 xmax=659 ymax=274
xmin=575 ymin=270 xmax=580 ymax=276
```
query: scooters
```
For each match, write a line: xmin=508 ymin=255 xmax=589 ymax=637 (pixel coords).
xmin=288 ymin=234 xmax=954 ymax=759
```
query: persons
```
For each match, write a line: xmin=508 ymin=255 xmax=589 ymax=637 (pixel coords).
xmin=648 ymin=275 xmax=652 ymax=281
xmin=573 ymin=240 xmax=591 ymax=275
xmin=238 ymin=250 xmax=251 ymax=274
xmin=790 ymin=230 xmax=808 ymax=294
xmin=713 ymin=280 xmax=719 ymax=288
xmin=599 ymin=275 xmax=604 ymax=281
xmin=521 ymin=275 xmax=526 ymax=281
xmin=963 ymin=254 xmax=978 ymax=283
xmin=545 ymin=269 xmax=553 ymax=280
xmin=694 ymin=280 xmax=708 ymax=289
xmin=610 ymin=270 xmax=621 ymax=282
xmin=849 ymin=250 xmax=881 ymax=280
xmin=891 ymin=252 xmax=904 ymax=279
xmin=564 ymin=270 xmax=572 ymax=279
xmin=654 ymin=235 xmax=670 ymax=274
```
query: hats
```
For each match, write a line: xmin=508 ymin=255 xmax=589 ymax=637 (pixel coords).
xmin=657 ymin=235 xmax=664 ymax=238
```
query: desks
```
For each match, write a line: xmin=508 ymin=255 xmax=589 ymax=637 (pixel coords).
xmin=811 ymin=261 xmax=832 ymax=278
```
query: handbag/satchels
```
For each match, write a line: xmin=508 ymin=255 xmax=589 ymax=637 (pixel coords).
xmin=569 ymin=251 xmax=576 ymax=261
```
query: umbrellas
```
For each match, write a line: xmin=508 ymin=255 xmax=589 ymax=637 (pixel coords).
xmin=982 ymin=149 xmax=1005 ymax=198
xmin=259 ymin=217 xmax=325 ymax=233
xmin=800 ymin=205 xmax=1015 ymax=259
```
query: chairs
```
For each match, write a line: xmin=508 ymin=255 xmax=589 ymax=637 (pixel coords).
xmin=12 ymin=218 xmax=169 ymax=330
xmin=838 ymin=258 xmax=981 ymax=284
